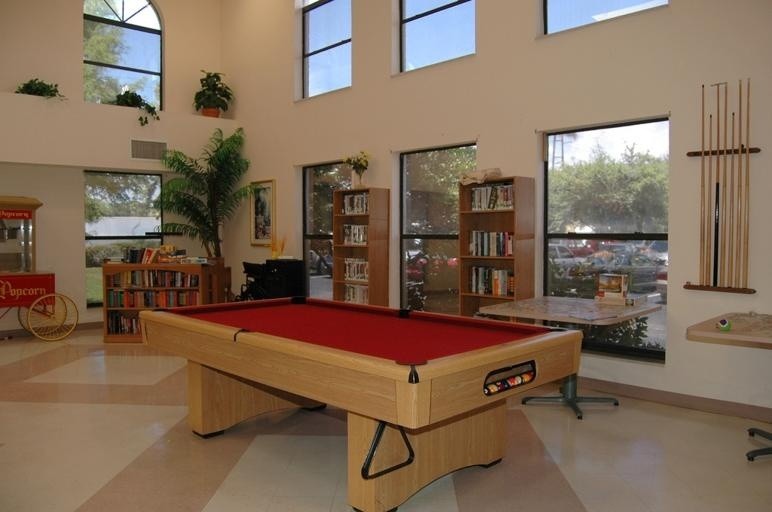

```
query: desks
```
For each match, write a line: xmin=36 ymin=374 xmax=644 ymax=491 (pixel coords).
xmin=478 ymin=296 xmax=662 ymax=418
xmin=685 ymin=313 xmax=772 ymax=461
xmin=139 ymin=296 xmax=583 ymax=512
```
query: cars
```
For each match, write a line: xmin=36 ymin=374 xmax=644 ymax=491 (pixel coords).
xmin=548 ymin=238 xmax=668 ymax=292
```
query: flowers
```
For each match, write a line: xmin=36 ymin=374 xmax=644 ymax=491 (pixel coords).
xmin=343 ymin=149 xmax=370 ymax=184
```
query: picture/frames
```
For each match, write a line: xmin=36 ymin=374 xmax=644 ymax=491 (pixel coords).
xmin=249 ymin=179 xmax=276 ymax=247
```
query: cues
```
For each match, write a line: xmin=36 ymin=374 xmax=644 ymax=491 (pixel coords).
xmin=698 ymin=79 xmax=750 ymax=287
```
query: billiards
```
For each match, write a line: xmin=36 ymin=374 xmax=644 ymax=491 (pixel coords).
xmin=718 ymin=319 xmax=731 ymax=331
xmin=484 ymin=371 xmax=533 ymax=396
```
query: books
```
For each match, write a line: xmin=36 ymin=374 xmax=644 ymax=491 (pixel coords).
xmin=121 ymin=246 xmax=207 ymax=265
xmin=467 ymin=229 xmax=517 ymax=296
xmin=103 ymin=269 xmax=204 ymax=339
xmin=341 ymin=193 xmax=370 ymax=304
xmin=472 ymin=183 xmax=515 ymax=210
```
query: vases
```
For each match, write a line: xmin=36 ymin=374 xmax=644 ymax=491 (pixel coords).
xmin=350 ymin=170 xmax=368 ymax=189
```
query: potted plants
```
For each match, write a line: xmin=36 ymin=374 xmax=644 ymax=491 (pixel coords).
xmin=153 ymin=125 xmax=261 ymax=289
xmin=193 ymin=69 xmax=233 ymax=118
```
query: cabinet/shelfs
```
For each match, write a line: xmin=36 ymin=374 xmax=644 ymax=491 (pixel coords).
xmin=458 ymin=177 xmax=536 ymax=324
xmin=102 ymin=263 xmax=218 ymax=342
xmin=332 ymin=188 xmax=389 ymax=306
